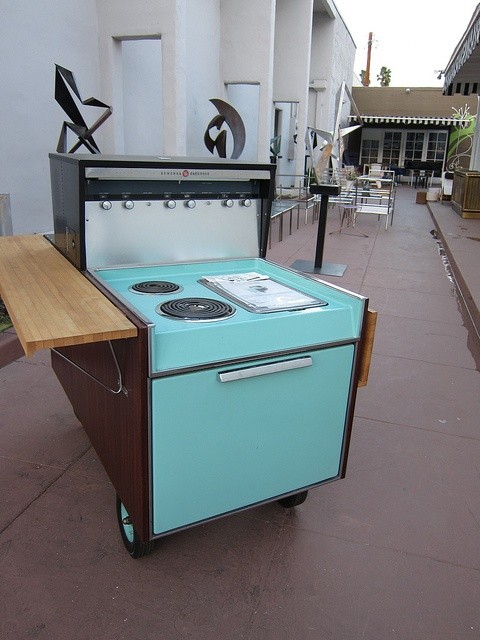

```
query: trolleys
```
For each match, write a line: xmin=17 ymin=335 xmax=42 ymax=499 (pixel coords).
xmin=0 ymin=136 xmax=383 ymax=561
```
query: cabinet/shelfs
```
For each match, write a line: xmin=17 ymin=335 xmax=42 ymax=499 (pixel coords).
xmin=352 ymin=170 xmax=396 ymax=231
xmin=274 ymin=174 xmax=322 ymax=224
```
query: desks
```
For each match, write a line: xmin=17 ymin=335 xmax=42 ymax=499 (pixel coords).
xmin=329 ymin=204 xmax=370 ymax=237
xmin=267 ymin=200 xmax=301 ymax=249
xmin=406 ymin=160 xmax=443 ymax=188
xmin=390 ymin=166 xmax=411 ymax=186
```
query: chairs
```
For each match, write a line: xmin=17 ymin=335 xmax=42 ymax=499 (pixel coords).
xmin=278 ymin=199 xmax=300 ymax=236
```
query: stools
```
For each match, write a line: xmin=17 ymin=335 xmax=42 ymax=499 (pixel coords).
xmin=415 ymin=176 xmax=428 ymax=189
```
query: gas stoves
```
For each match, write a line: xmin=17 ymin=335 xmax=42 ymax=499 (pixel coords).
xmin=85 ymin=255 xmax=369 ymax=380
